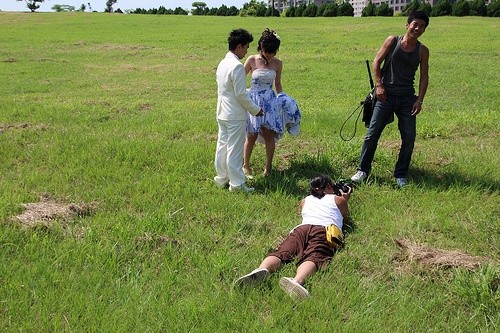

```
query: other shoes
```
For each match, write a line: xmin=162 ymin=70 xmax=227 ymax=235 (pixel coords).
xmin=233 ymin=269 xmax=269 ymax=291
xmin=397 ymin=177 xmax=408 ymax=188
xmin=243 ymin=166 xmax=253 ymax=179
xmin=217 ymin=182 xmax=229 ymax=189
xmin=278 ymin=277 xmax=310 ymax=304
xmin=264 ymin=165 xmax=273 ymax=178
xmin=229 ymin=184 xmax=255 ymax=192
xmin=351 ymin=170 xmax=367 ymax=184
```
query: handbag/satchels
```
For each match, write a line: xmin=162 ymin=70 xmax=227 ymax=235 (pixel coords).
xmin=362 ymin=92 xmax=394 ymax=128
xmin=326 ymin=224 xmax=345 ymax=249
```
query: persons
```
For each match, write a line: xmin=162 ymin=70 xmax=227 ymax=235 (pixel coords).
xmin=214 ymin=28 xmax=264 ymax=192
xmin=241 ymin=28 xmax=282 ymax=180
xmin=235 ymin=174 xmax=351 ymax=301
xmin=351 ymin=11 xmax=429 ymax=185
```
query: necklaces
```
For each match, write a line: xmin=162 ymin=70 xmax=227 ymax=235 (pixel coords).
xmin=259 ymin=52 xmax=273 ymax=66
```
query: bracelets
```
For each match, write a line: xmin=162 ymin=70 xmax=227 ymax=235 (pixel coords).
xmin=417 ymin=100 xmax=423 ymax=104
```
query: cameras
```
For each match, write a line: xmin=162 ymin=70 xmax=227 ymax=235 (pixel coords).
xmin=333 ymin=179 xmax=353 ymax=195
